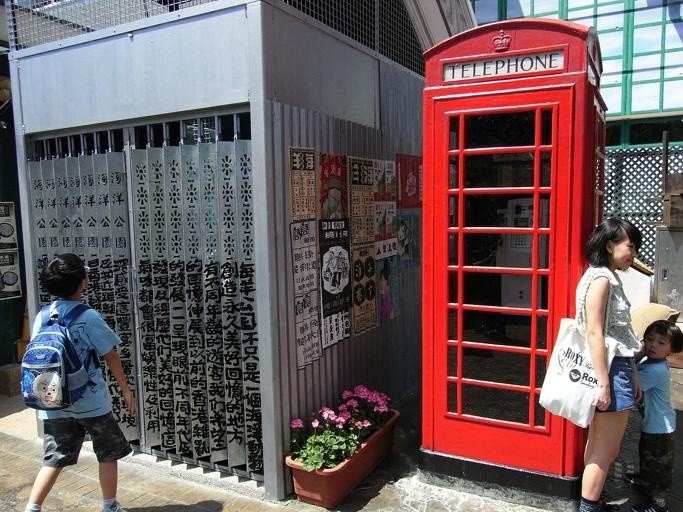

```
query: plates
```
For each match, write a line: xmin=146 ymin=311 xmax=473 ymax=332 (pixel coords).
xmin=654 ymin=226 xmax=682 ymax=317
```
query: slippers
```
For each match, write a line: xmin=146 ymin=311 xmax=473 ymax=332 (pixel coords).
xmin=20 ymin=304 xmax=94 ymax=411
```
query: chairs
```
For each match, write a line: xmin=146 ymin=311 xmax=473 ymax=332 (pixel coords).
xmin=631 ymin=501 xmax=669 ymax=512
xmin=622 ymin=468 xmax=653 ymax=489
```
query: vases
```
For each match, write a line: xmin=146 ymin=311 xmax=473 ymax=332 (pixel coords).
xmin=290 ymin=381 xmax=392 ymax=473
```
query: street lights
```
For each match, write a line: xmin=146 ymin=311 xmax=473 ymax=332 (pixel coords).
xmin=535 ymin=275 xmax=620 ymax=432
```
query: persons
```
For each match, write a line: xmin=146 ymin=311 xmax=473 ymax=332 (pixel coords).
xmin=20 ymin=252 xmax=138 ymax=512
xmin=632 ymin=316 xmax=681 ymax=511
xmin=572 ymin=216 xmax=646 ymax=511
xmin=379 ymin=258 xmax=395 ymax=321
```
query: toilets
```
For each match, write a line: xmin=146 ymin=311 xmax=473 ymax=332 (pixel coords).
xmin=495 ymin=198 xmax=547 ymax=268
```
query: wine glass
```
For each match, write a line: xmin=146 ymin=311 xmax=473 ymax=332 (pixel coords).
xmin=604 ymin=400 xmax=607 ymax=403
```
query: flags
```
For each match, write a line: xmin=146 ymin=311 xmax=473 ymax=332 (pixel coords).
xmin=283 ymin=409 xmax=401 ymax=511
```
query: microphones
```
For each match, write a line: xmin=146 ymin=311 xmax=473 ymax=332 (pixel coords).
xmin=0 ymin=364 xmax=26 ymax=398
